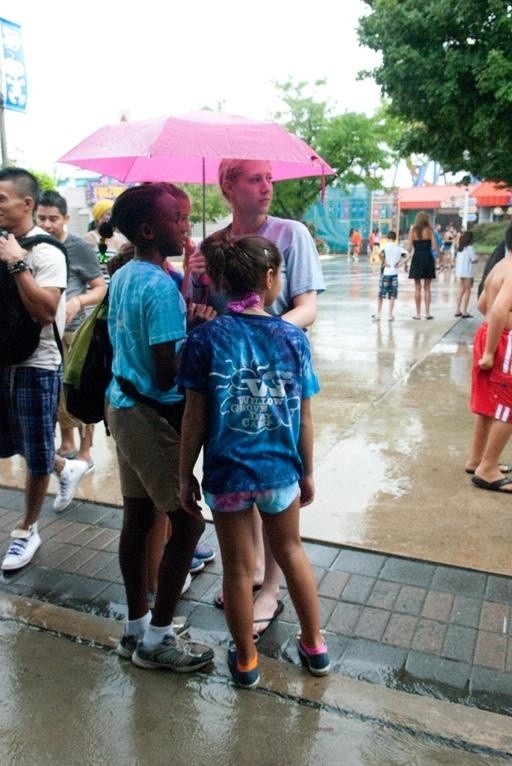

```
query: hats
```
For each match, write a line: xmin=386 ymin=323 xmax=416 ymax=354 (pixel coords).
xmin=93 ymin=198 xmax=114 ymax=220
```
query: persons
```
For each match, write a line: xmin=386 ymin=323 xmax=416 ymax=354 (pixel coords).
xmin=84 ymin=200 xmax=131 ymax=314
xmin=182 ymin=142 xmax=326 ymax=646
xmin=177 ymin=235 xmax=332 ymax=689
xmin=477 ymin=225 xmax=508 ymax=295
xmin=454 ymin=232 xmax=482 ymax=319
xmin=404 ymin=211 xmax=443 ymax=320
xmin=372 ymin=229 xmax=380 ymax=262
xmin=464 ymin=224 xmax=512 ymax=495
xmin=431 ymin=223 xmax=442 ymax=261
xmin=0 ymin=167 xmax=88 ymax=573
xmin=348 ymin=228 xmax=361 ymax=257
xmin=371 ymin=231 xmax=409 ymax=322
xmin=98 ymin=186 xmax=215 ymax=673
xmin=447 ymin=221 xmax=456 ymax=266
xmin=34 ymin=190 xmax=107 ymax=479
xmin=110 ymin=182 xmax=214 ymax=574
xmin=379 ymin=233 xmax=397 ymax=298
xmin=442 ymin=226 xmax=455 ymax=268
xmin=368 ymin=230 xmax=376 ymax=251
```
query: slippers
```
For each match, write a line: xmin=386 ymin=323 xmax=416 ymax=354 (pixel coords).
xmin=213 ymin=581 xmax=261 ymax=609
xmin=252 ymin=599 xmax=283 ymax=640
xmin=466 ymin=462 xmax=512 ymax=473
xmin=469 ymin=473 xmax=512 ymax=493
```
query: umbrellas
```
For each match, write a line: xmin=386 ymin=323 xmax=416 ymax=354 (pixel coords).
xmin=55 ymin=115 xmax=338 ymax=208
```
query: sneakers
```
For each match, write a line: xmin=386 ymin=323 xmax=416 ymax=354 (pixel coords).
xmin=1 ymin=520 xmax=41 ymax=571
xmin=194 ymin=545 xmax=216 ymax=562
xmin=132 ymin=634 xmax=214 ymax=674
xmin=188 ymin=558 xmax=205 ymax=574
xmin=116 ymin=616 xmax=190 ymax=657
xmin=227 ymin=641 xmax=261 ymax=691
xmin=295 ymin=630 xmax=333 ymax=677
xmin=53 ymin=447 xmax=78 ymax=472
xmin=52 ymin=458 xmax=88 ymax=513
xmin=83 ymin=456 xmax=95 ymax=475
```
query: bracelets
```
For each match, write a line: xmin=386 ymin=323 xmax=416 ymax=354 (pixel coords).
xmin=74 ymin=295 xmax=84 ymax=313
xmin=8 ymin=261 xmax=27 ymax=274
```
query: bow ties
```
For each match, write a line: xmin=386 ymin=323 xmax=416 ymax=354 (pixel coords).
xmin=227 ymin=292 xmax=260 ymax=313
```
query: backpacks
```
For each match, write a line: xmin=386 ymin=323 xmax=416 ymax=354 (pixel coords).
xmin=0 ymin=234 xmax=71 ymax=368
